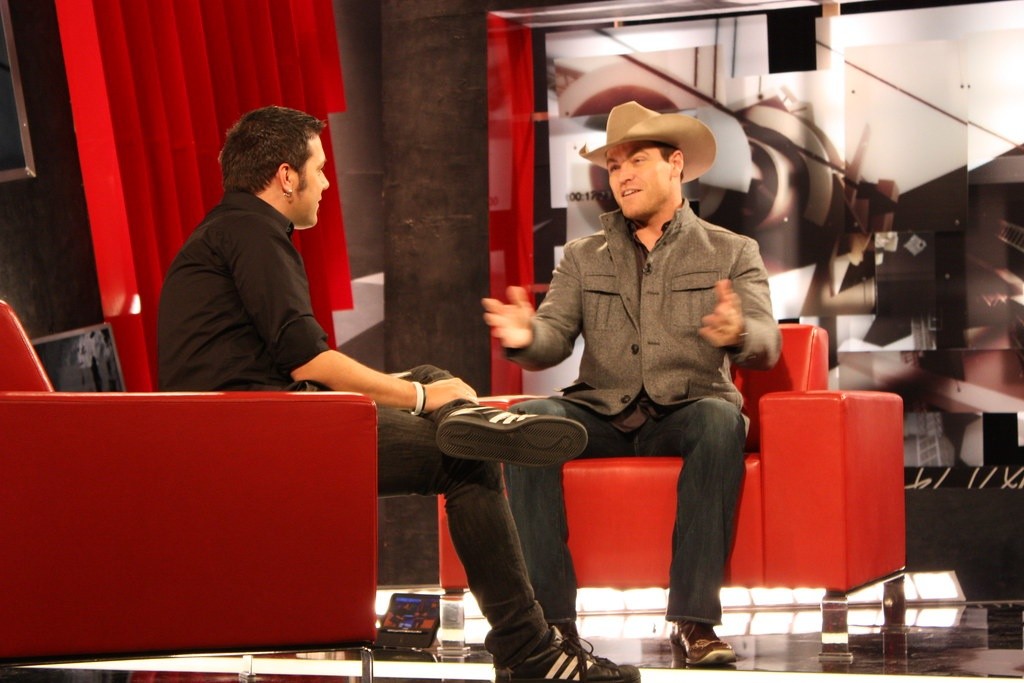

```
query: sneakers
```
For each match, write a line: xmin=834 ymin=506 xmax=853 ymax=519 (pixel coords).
xmin=436 ymin=405 xmax=589 ymax=468
xmin=494 ymin=625 xmax=642 ymax=683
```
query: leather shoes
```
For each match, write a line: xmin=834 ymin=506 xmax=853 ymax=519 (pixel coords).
xmin=669 ymin=621 xmax=737 ymax=668
xmin=550 ymin=621 xmax=577 ymax=638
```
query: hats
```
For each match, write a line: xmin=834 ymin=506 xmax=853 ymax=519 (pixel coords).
xmin=578 ymin=100 xmax=720 ymax=184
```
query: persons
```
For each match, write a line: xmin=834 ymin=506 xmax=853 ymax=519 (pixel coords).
xmin=156 ymin=105 xmax=644 ymax=682
xmin=484 ymin=100 xmax=783 ymax=663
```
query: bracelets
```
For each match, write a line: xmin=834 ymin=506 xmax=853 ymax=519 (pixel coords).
xmin=411 ymin=381 xmax=426 ymax=415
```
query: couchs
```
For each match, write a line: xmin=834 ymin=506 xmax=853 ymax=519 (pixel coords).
xmin=559 ymin=323 xmax=906 ymax=661
xmin=0 ymin=300 xmax=379 ymax=683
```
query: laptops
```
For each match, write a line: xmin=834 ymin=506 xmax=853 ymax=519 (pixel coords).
xmin=366 ymin=592 xmax=441 ymax=650
